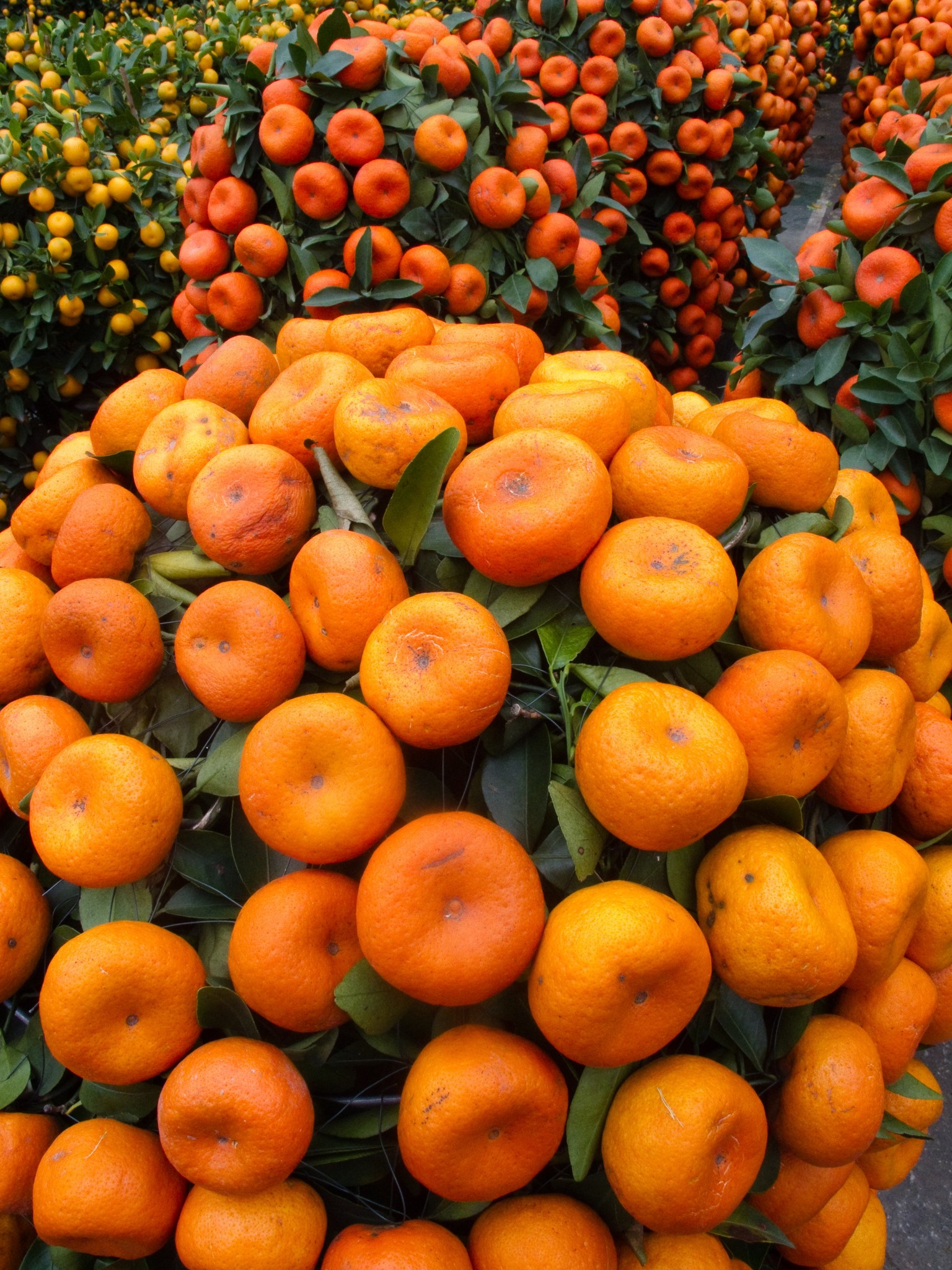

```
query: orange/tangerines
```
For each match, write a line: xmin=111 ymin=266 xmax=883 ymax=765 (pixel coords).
xmin=1 ymin=0 xmax=952 ymax=1269
